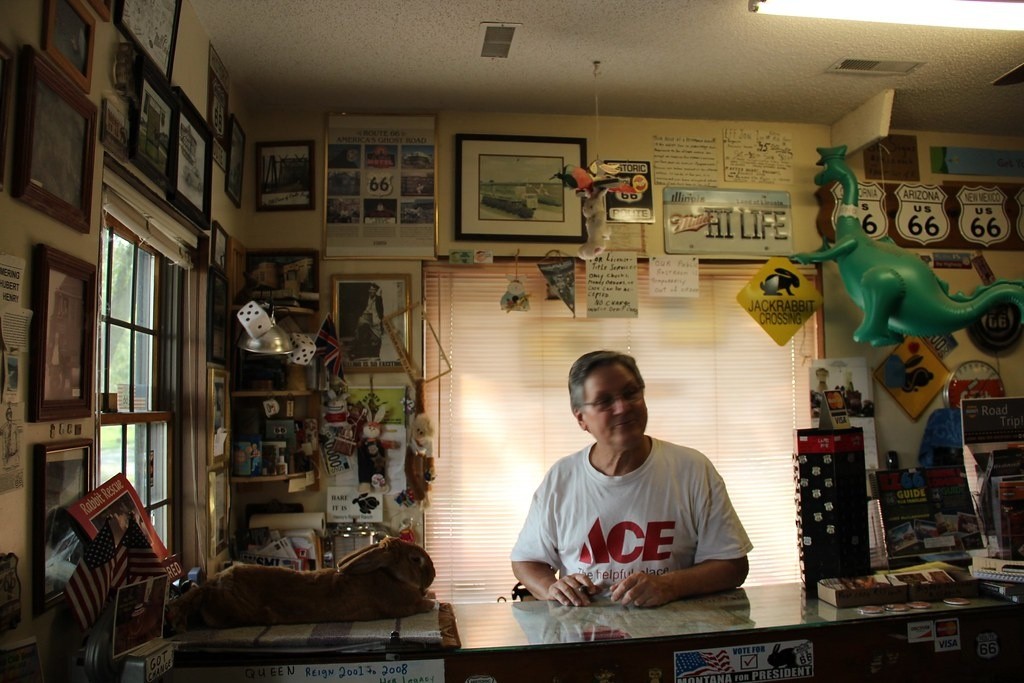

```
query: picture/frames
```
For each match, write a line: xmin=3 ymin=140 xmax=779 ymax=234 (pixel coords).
xmin=331 ymin=272 xmax=412 ymax=374
xmin=455 ymin=133 xmax=587 ymax=244
xmin=254 ymin=139 xmax=316 ymax=212
xmin=12 ymin=0 xmax=246 ymax=619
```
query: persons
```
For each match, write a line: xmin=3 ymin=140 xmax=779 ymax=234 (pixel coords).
xmin=513 ymin=602 xmax=755 ymax=644
xmin=355 ymin=282 xmax=384 ymax=358
xmin=510 ymin=350 xmax=754 ymax=607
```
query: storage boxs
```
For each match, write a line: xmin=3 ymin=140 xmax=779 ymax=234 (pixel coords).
xmin=817 ymin=571 xmax=972 ymax=609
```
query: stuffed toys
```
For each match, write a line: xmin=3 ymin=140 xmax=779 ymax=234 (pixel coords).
xmin=577 ymin=187 xmax=612 ymax=260
xmin=405 ymin=413 xmax=435 ymax=512
xmin=361 ymin=403 xmax=386 ymax=467
xmin=170 ymin=535 xmax=436 ymax=634
xmin=322 ymin=388 xmax=350 ymax=427
xmin=500 ymin=274 xmax=529 ymax=311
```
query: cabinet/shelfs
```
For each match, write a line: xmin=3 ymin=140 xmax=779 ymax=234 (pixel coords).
xmin=224 ymin=233 xmax=321 ymax=490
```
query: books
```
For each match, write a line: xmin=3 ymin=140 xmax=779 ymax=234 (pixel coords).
xmin=991 ymin=475 xmax=1024 ymax=548
xmin=968 ymin=556 xmax=1024 ymax=604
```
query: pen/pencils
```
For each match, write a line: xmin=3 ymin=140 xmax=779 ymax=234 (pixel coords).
xmin=579 ymin=585 xmax=588 ymax=592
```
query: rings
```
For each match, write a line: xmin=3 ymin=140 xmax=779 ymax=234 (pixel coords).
xmin=578 ymin=586 xmax=588 ymax=594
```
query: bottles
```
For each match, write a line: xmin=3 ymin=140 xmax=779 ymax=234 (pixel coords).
xmin=232 ymin=435 xmax=287 ymax=477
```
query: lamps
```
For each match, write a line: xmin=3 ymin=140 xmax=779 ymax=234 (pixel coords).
xmin=238 ymin=278 xmax=316 ymax=365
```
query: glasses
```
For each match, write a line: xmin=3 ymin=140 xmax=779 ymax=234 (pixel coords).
xmin=582 ymin=380 xmax=646 ymax=411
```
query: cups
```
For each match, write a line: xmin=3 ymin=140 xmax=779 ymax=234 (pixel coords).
xmin=99 ymin=393 xmax=118 ymax=410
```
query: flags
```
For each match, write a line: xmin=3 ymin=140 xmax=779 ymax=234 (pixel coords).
xmin=63 ymin=518 xmax=117 ymax=633
xmin=111 ymin=517 xmax=167 ymax=594
xmin=315 ymin=314 xmax=348 ymax=385
